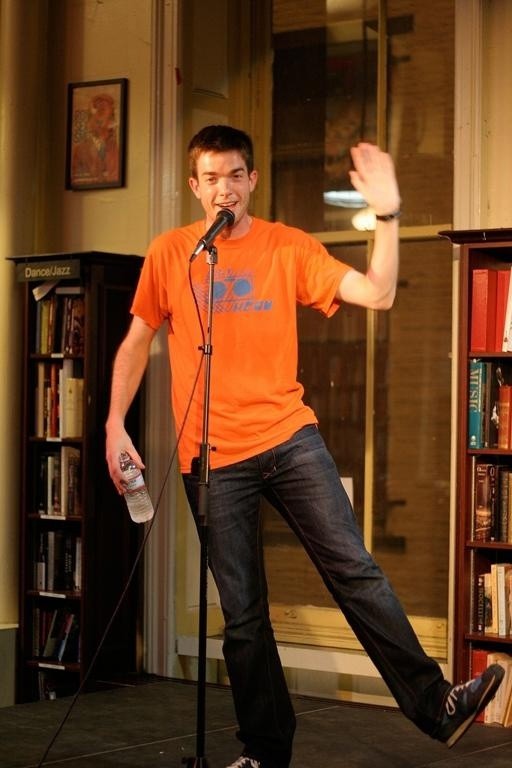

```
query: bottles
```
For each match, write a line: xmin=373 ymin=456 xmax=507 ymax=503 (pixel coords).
xmin=119 ymin=453 xmax=155 ymax=523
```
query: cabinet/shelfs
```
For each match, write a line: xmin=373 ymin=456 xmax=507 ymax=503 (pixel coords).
xmin=4 ymin=250 xmax=147 ymax=699
xmin=438 ymin=226 xmax=512 ymax=730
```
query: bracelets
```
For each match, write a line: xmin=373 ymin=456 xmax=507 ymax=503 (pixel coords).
xmin=374 ymin=209 xmax=402 ymax=225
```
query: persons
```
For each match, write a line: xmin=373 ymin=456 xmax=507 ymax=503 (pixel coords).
xmin=106 ymin=123 xmax=506 ymax=768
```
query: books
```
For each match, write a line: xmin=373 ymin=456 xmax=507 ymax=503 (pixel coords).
xmin=19 ymin=274 xmax=88 ymax=699
xmin=460 ymin=263 xmax=511 ymax=726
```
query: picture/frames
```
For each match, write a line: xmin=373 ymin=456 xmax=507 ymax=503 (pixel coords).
xmin=65 ymin=77 xmax=128 ymax=192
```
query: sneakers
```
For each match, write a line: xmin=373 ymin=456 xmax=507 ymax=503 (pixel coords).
xmin=226 ymin=755 xmax=265 ymax=768
xmin=437 ymin=664 xmax=504 ymax=749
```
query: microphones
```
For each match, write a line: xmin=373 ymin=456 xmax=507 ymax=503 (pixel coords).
xmin=189 ymin=207 xmax=235 ymax=263
